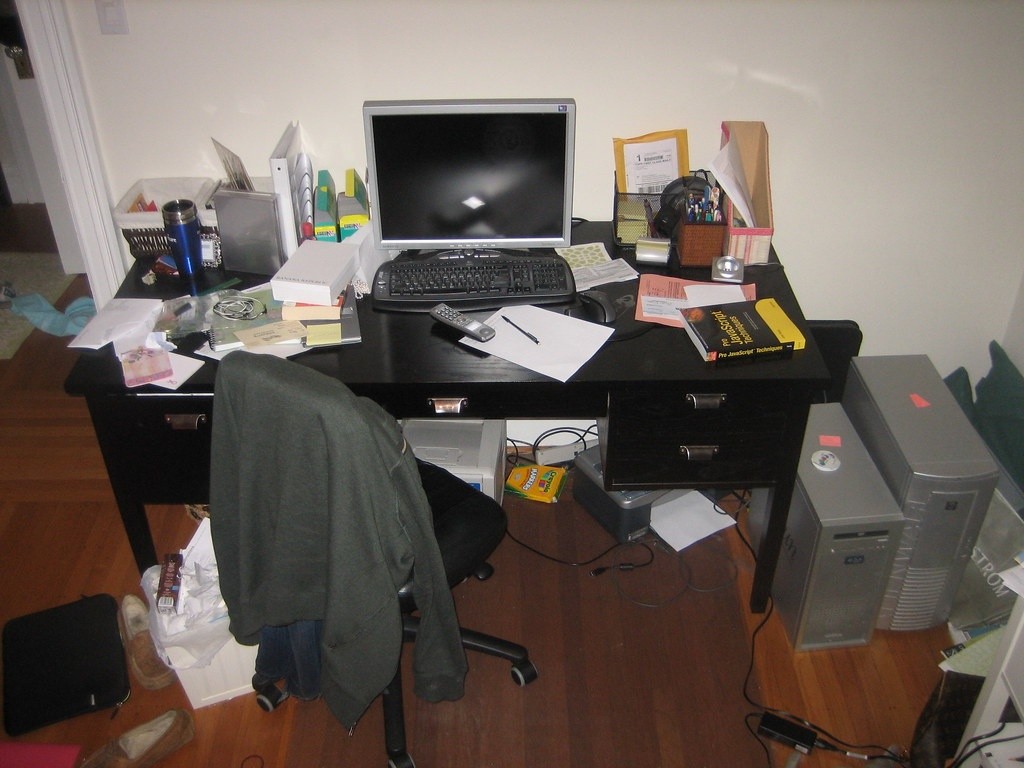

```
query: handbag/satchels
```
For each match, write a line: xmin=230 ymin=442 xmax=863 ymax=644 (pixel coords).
xmin=0 ymin=592 xmax=132 ymax=736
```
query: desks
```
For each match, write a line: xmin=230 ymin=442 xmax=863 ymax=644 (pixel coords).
xmin=64 ymin=220 xmax=830 ymax=614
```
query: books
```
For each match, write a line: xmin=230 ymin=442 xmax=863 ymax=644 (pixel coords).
xmin=678 ymin=298 xmax=807 ymax=363
xmin=205 ymin=238 xmax=361 ymax=353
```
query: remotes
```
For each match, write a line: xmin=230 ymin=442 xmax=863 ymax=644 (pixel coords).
xmin=431 ymin=303 xmax=496 ymax=342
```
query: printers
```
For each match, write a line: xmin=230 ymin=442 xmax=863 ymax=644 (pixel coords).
xmin=401 ymin=417 xmax=507 ymax=505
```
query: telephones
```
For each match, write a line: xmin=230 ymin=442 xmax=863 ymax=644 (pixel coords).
xmin=428 ymin=302 xmax=496 ymax=343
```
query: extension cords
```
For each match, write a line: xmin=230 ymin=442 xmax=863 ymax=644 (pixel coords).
xmin=535 ymin=439 xmax=599 ymax=465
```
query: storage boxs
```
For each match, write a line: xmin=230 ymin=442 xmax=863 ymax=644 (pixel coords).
xmin=398 ymin=418 xmax=508 ymax=510
xmin=613 ymin=171 xmax=719 ymax=248
xmin=141 ymin=565 xmax=261 ymax=712
xmin=111 ymin=177 xmax=275 ymax=259
xmin=313 ymin=169 xmax=338 ymax=243
xmin=337 ymin=169 xmax=370 ymax=242
xmin=720 ymin=120 xmax=774 ymax=265
xmin=675 ymin=211 xmax=728 ymax=265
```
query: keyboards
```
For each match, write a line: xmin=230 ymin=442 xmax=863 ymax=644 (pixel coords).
xmin=371 ymin=248 xmax=576 ymax=312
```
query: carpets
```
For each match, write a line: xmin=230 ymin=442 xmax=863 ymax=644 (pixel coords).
xmin=0 ymin=252 xmax=79 ymax=361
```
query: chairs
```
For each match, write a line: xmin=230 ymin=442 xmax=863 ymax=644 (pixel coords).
xmin=208 ymin=356 xmax=540 ymax=768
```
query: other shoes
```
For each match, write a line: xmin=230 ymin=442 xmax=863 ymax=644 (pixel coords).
xmin=117 ymin=594 xmax=177 ymax=690
xmin=75 ymin=708 xmax=194 ymax=768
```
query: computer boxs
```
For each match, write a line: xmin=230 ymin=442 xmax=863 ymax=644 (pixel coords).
xmin=749 ymin=353 xmax=998 ymax=651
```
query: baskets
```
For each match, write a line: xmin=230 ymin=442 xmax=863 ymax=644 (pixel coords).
xmin=195 ymin=176 xmax=224 ymax=268
xmin=112 ymin=176 xmax=214 ymax=259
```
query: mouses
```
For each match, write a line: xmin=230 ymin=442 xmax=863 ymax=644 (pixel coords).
xmin=580 ymin=290 xmax=616 ymax=323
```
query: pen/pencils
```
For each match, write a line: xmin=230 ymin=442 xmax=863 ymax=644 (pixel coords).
xmin=688 ymin=192 xmax=723 ymax=223
xmin=501 ymin=314 xmax=539 ymax=345
xmin=643 ymin=198 xmax=658 ymax=237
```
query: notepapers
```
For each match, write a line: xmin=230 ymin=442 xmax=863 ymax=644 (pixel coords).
xmin=306 ymin=322 xmax=342 ymax=346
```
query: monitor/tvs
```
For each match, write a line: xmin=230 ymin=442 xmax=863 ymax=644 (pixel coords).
xmin=361 ymin=96 xmax=576 ymax=255
